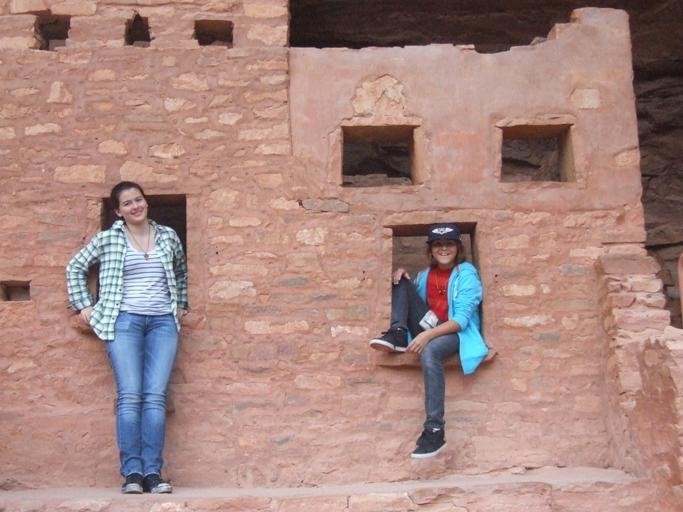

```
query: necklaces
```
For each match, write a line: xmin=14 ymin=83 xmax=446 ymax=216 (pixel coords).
xmin=431 ymin=266 xmax=449 ymax=296
xmin=121 ymin=222 xmax=152 ymax=262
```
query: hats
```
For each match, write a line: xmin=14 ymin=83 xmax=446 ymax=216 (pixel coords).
xmin=425 ymin=223 xmax=462 ymax=243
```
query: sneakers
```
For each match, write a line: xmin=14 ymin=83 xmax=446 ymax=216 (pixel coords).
xmin=368 ymin=326 xmax=408 ymax=353
xmin=120 ymin=474 xmax=144 ymax=494
xmin=145 ymin=475 xmax=172 ymax=494
xmin=410 ymin=426 xmax=449 ymax=459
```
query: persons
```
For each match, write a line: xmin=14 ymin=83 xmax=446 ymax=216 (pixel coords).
xmin=62 ymin=180 xmax=189 ymax=496
xmin=366 ymin=224 xmax=489 ymax=460
